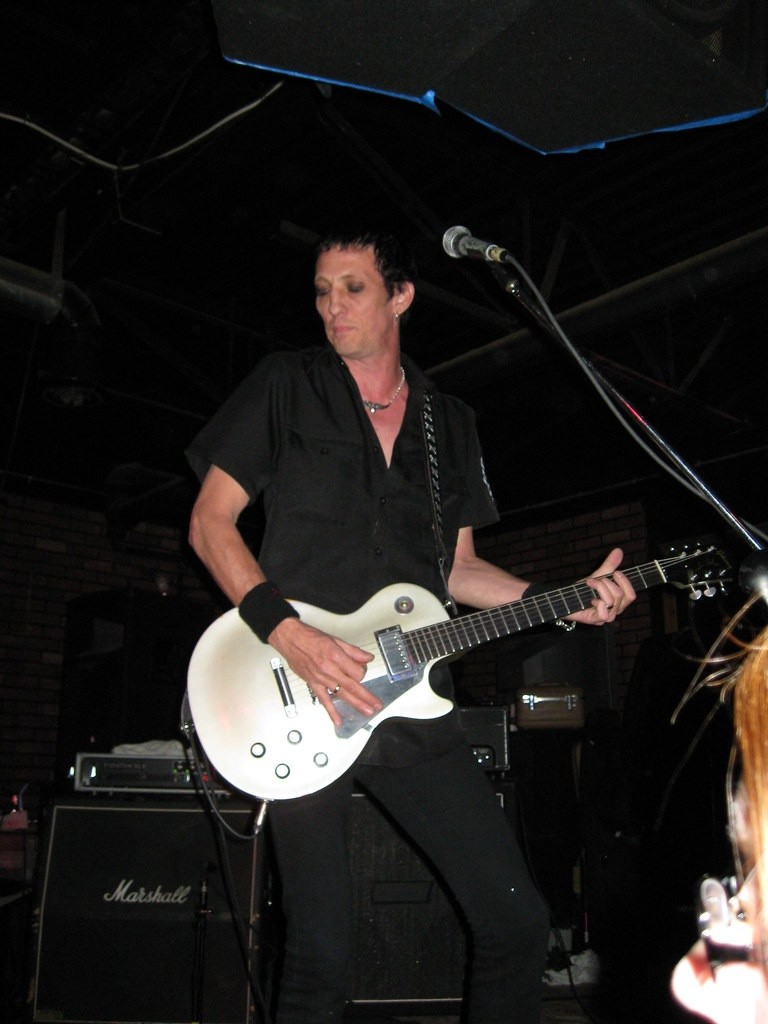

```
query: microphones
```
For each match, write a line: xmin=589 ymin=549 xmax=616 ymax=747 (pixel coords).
xmin=442 ymin=226 xmax=511 ymax=265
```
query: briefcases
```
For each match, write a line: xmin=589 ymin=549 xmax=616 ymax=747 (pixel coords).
xmin=518 ymin=687 xmax=584 ymax=728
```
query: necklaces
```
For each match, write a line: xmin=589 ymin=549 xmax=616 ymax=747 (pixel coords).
xmin=361 ymin=366 xmax=405 ymax=413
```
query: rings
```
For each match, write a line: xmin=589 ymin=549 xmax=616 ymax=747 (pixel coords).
xmin=333 ymin=684 xmax=340 ymax=694
xmin=607 ymin=606 xmax=613 ymax=609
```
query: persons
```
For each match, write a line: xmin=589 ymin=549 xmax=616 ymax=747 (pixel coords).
xmin=667 ymin=628 xmax=768 ymax=1024
xmin=185 ymin=213 xmax=638 ymax=1024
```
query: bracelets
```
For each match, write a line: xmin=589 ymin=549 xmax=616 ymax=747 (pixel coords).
xmin=239 ymin=580 xmax=300 ymax=644
xmin=523 ymin=582 xmax=577 ymax=631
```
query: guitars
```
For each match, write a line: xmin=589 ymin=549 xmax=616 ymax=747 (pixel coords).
xmin=188 ymin=544 xmax=739 ymax=802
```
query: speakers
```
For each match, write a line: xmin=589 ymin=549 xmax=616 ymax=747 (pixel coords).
xmin=29 ymin=801 xmax=268 ymax=1024
xmin=343 ymin=782 xmax=519 ymax=1016
